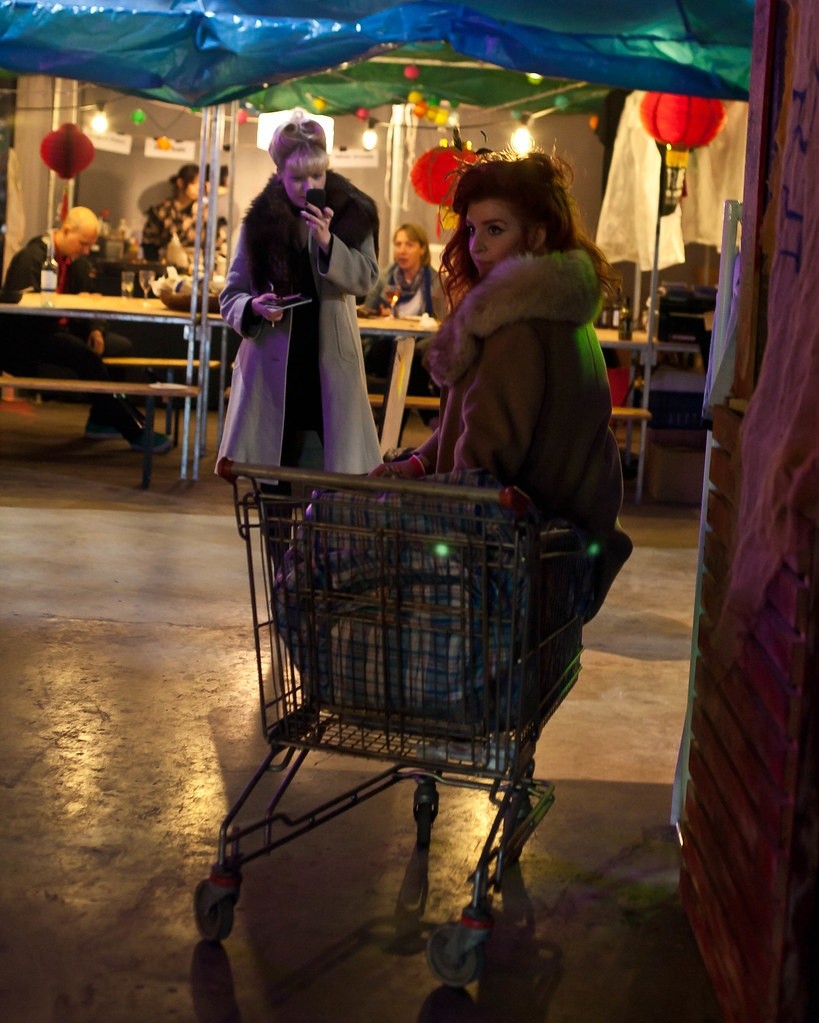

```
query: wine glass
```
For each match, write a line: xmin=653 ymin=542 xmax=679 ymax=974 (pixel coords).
xmin=139 ymin=270 xmax=154 ymax=306
xmin=386 ymin=285 xmax=400 ymax=322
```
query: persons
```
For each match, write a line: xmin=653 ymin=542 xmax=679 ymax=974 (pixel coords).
xmin=214 ymin=118 xmax=382 ymax=577
xmin=364 ymin=224 xmax=448 ymax=447
xmin=367 ymin=153 xmax=623 ymax=548
xmin=200 ymin=164 xmax=239 ymax=230
xmin=143 ymin=165 xmax=225 ymax=274
xmin=0 ymin=206 xmax=168 ymax=452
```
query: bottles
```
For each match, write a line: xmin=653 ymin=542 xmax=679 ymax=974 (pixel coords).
xmin=99 ymin=209 xmax=111 ymax=237
xmin=41 ymin=242 xmax=58 ymax=304
xmin=600 ymin=294 xmax=610 ymax=328
xmin=117 ymin=219 xmax=129 ymax=239
xmin=619 ymin=305 xmax=632 ymax=340
xmin=613 ymin=299 xmax=619 ymax=328
xmin=664 ymin=412 xmax=702 ymax=430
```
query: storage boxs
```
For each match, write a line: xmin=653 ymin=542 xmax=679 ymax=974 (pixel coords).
xmin=645 ymin=425 xmax=707 ymax=503
xmin=633 ymin=387 xmax=712 ymax=429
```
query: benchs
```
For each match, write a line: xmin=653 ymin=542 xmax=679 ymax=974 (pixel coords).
xmin=101 ymin=356 xmax=222 ymax=446
xmin=225 ymin=387 xmax=384 ymax=409
xmin=406 ymin=397 xmax=653 ymax=462
xmin=0 ymin=375 xmax=204 ymax=490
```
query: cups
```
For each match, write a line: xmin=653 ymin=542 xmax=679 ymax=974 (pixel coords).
xmin=120 ymin=272 xmax=135 ymax=304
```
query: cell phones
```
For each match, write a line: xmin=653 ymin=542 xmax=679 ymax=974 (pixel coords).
xmin=305 ymin=188 xmax=326 ymax=220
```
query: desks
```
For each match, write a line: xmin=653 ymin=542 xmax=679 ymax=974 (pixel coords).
xmin=199 ymin=308 xmax=700 ymax=459
xmin=0 ymin=290 xmax=199 ymax=482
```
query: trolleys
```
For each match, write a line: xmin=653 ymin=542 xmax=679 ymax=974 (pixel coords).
xmin=191 ymin=457 xmax=586 ymax=989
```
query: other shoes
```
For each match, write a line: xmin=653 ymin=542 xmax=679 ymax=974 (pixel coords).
xmin=130 ymin=428 xmax=170 ymax=454
xmin=84 ymin=412 xmax=123 ymax=437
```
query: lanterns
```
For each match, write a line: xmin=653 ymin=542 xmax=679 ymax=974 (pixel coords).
xmin=39 ymin=122 xmax=95 ymax=219
xmin=410 ymin=146 xmax=479 ymax=231
xmin=639 ymin=92 xmax=724 ymax=168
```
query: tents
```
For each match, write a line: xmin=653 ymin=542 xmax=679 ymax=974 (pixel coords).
xmin=0 ymin=0 xmax=758 ymax=481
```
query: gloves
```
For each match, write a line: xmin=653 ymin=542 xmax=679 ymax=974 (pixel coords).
xmin=365 ymin=455 xmax=425 ymax=480
